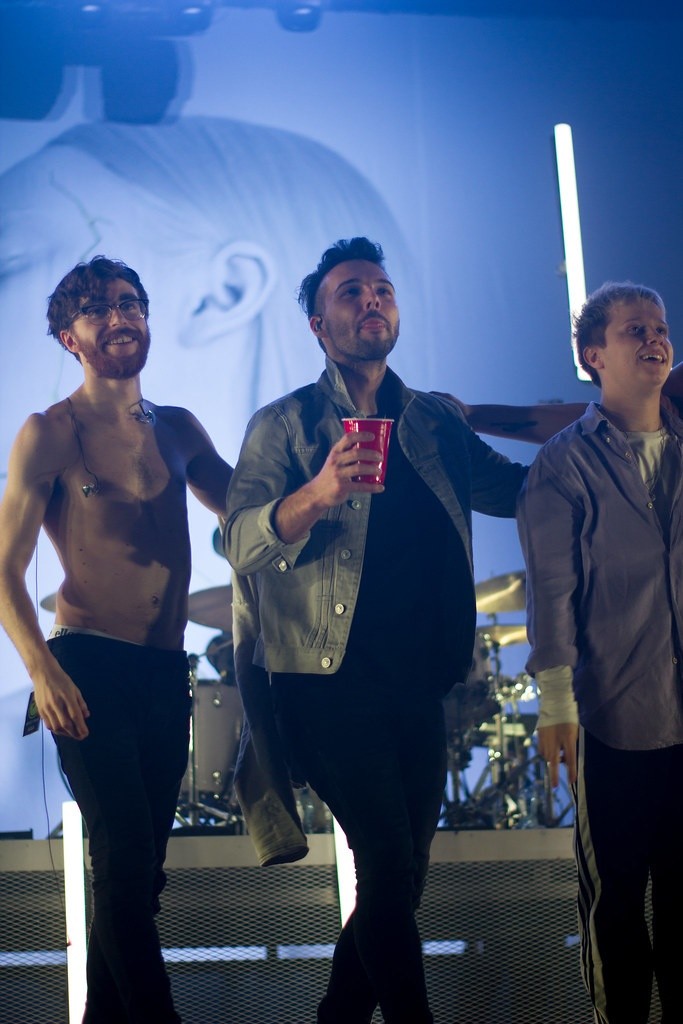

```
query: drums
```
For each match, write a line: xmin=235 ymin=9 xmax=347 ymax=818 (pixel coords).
xmin=178 ymin=677 xmax=250 ymax=817
xmin=443 ymin=630 xmax=505 ymax=733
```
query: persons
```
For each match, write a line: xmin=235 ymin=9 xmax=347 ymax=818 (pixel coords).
xmin=0 ymin=252 xmax=231 ymax=1024
xmin=224 ymin=235 xmax=531 ymax=1024
xmin=513 ymin=281 xmax=683 ymax=1024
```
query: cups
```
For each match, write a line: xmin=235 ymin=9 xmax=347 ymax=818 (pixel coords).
xmin=342 ymin=417 xmax=394 ymax=486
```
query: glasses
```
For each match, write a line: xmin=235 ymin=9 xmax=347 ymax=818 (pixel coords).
xmin=65 ymin=298 xmax=148 ymax=328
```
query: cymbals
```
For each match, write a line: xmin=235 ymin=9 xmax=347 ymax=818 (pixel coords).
xmin=478 ymin=621 xmax=529 ymax=648
xmin=38 ymin=592 xmax=55 ymax=614
xmin=475 ymin=568 xmax=528 ymax=615
xmin=183 ymin=584 xmax=233 ymax=633
